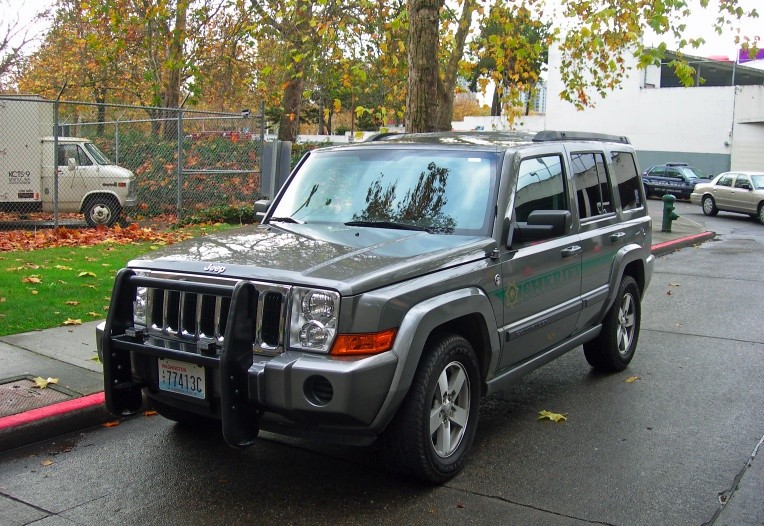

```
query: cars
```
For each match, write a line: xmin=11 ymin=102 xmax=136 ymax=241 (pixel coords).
xmin=688 ymin=170 xmax=764 ymax=226
xmin=642 ymin=162 xmax=714 ymax=205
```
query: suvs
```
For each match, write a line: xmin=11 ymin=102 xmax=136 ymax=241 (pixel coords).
xmin=94 ymin=129 xmax=659 ymax=487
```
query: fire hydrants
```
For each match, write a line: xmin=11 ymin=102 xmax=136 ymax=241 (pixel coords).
xmin=661 ymin=193 xmax=680 ymax=233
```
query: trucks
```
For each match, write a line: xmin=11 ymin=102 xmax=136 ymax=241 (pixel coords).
xmin=0 ymin=92 xmax=138 ymax=228
